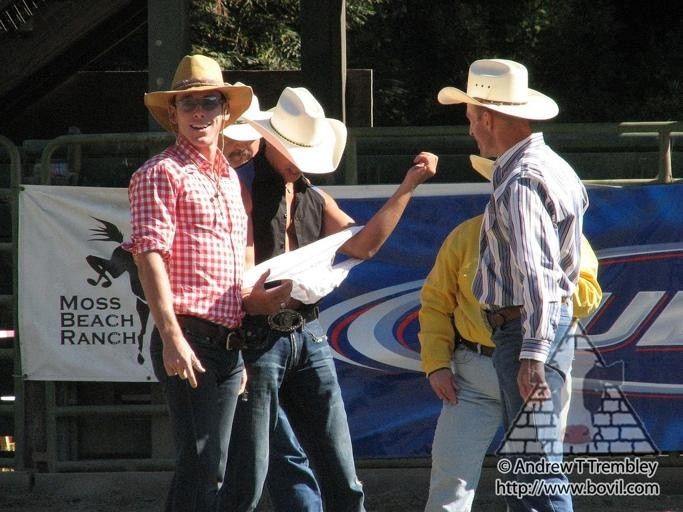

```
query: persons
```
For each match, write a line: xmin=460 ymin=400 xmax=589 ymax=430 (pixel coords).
xmin=417 ymin=155 xmax=604 ymax=512
xmin=233 ymin=87 xmax=441 ymax=512
xmin=217 ymin=79 xmax=326 ymax=512
xmin=119 ymin=54 xmax=256 ymax=512
xmin=436 ymin=56 xmax=591 ymax=512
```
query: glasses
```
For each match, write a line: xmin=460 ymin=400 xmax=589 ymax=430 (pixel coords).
xmin=171 ymin=96 xmax=224 ymax=111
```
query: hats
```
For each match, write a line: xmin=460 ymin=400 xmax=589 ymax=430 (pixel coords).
xmin=469 ymin=154 xmax=495 ymax=182
xmin=144 ymin=54 xmax=253 ymax=133
xmin=221 ymin=83 xmax=348 ymax=174
xmin=437 ymin=59 xmax=559 ymax=121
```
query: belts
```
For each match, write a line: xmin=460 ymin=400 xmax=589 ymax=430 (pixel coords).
xmin=481 ymin=306 xmax=526 ymax=332
xmin=178 ymin=316 xmax=245 ymax=350
xmin=242 ymin=308 xmax=317 ymax=332
xmin=458 ymin=338 xmax=494 ymax=357
